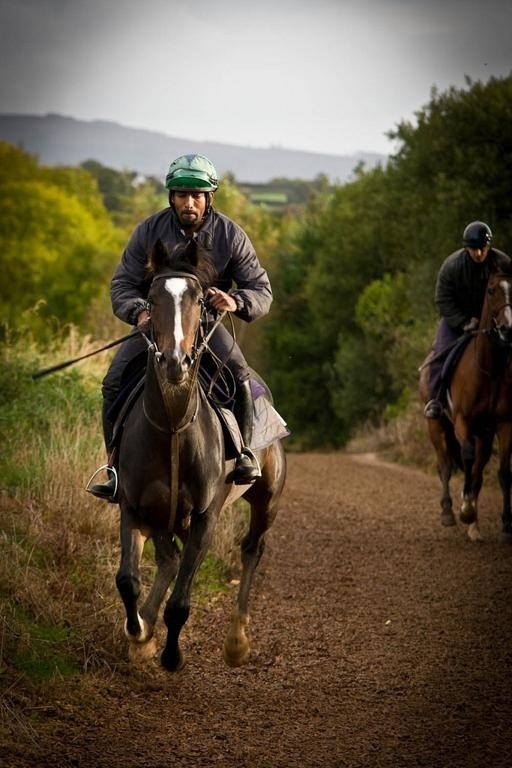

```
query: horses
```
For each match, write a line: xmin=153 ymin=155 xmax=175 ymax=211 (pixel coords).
xmin=418 ymin=260 xmax=512 ymax=546
xmin=115 ymin=235 xmax=289 ymax=671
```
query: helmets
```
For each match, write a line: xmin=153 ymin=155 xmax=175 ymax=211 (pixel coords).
xmin=462 ymin=220 xmax=493 ymax=251
xmin=164 ymin=153 xmax=220 ymax=193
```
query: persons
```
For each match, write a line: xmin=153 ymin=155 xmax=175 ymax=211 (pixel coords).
xmin=421 ymin=220 xmax=512 ymax=422
xmin=90 ymin=153 xmax=274 ymax=503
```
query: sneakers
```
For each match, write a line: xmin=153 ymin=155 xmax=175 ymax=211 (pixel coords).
xmin=91 ymin=478 xmax=120 ymax=499
xmin=236 ymin=459 xmax=259 ymax=485
xmin=423 ymin=402 xmax=444 ymax=420
